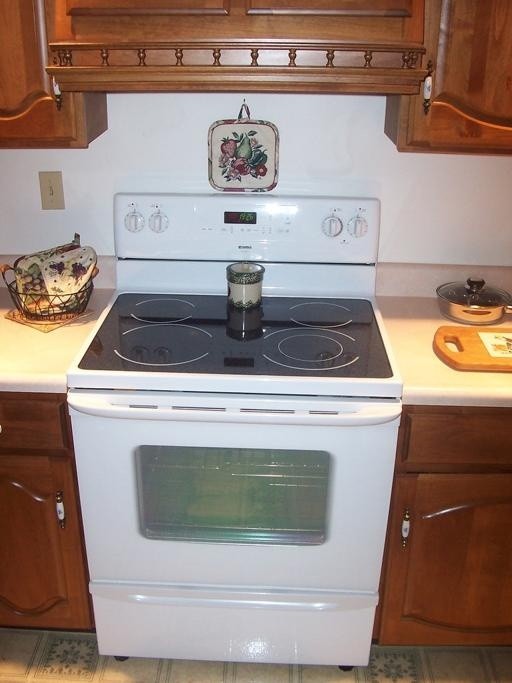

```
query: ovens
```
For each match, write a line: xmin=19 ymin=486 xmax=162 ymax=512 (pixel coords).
xmin=61 ymin=389 xmax=408 ymax=671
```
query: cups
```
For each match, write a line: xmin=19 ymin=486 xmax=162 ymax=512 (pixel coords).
xmin=225 ymin=261 xmax=266 ymax=309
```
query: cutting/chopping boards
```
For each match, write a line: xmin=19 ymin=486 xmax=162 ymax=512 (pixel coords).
xmin=432 ymin=325 xmax=512 ymax=370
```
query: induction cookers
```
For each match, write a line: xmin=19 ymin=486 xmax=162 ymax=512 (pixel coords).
xmin=66 ymin=256 xmax=403 ymax=401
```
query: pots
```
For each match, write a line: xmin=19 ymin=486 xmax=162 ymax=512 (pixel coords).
xmin=433 ymin=276 xmax=512 ymax=325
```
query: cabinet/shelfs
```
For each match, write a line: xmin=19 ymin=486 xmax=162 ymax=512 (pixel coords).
xmin=373 ymin=403 xmax=510 ymax=656
xmin=1 ymin=391 xmax=121 ymax=636
xmin=0 ymin=0 xmax=111 ymax=154
xmin=375 ymin=0 xmax=511 ymax=156
xmin=33 ymin=0 xmax=441 ymax=118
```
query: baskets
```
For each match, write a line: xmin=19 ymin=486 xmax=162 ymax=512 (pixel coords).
xmin=0 ymin=264 xmax=98 ymax=322
